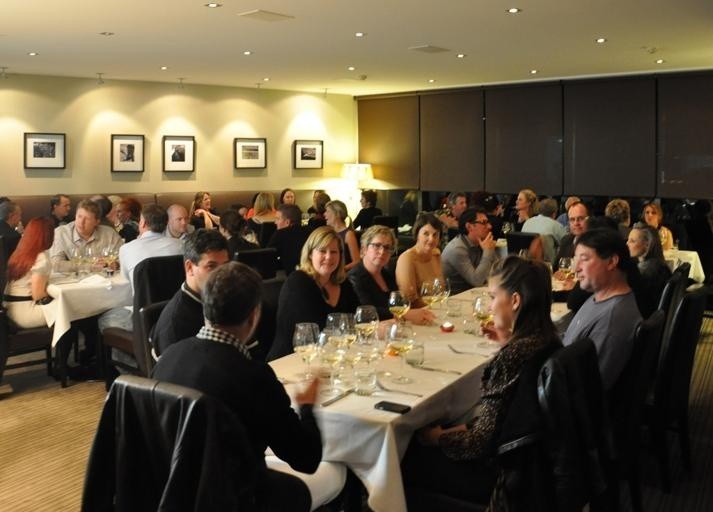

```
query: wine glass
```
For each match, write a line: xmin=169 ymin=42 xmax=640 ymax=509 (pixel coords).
xmin=50 ymin=244 xmax=122 ymax=285
xmin=675 ymin=198 xmax=698 ymax=219
xmin=290 ymin=276 xmax=494 ymax=410
xmin=517 ymin=248 xmax=578 ymax=284
xmin=300 ymin=212 xmax=317 ymax=226
xmin=500 ymin=219 xmax=511 ymax=238
xmin=243 ymin=228 xmax=256 ymax=244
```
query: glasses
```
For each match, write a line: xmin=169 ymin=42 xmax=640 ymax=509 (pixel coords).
xmin=473 ymin=219 xmax=490 ymax=224
xmin=367 ymin=242 xmax=396 ymax=254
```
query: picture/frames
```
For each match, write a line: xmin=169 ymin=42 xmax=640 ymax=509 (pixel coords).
xmin=109 ymin=134 xmax=145 ymax=173
xmin=233 ymin=137 xmax=267 ymax=170
xmin=293 ymin=140 xmax=323 ymax=169
xmin=161 ymin=135 xmax=195 ymax=173
xmin=23 ymin=132 xmax=66 ymax=170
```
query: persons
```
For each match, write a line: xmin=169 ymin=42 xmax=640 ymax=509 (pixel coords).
xmin=0 ymin=186 xmax=677 ymax=512
